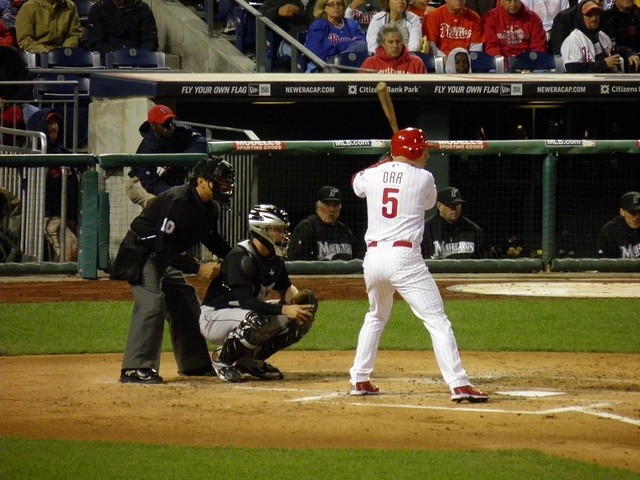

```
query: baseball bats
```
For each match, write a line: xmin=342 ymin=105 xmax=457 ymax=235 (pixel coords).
xmin=376 ymin=81 xmax=399 ymax=134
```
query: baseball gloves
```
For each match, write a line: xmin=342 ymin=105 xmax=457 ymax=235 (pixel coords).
xmin=289 ymin=289 xmax=318 ymax=335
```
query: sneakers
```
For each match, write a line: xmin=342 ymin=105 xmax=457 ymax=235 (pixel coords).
xmin=241 ymin=359 xmax=284 ymax=381
xmin=349 ymin=380 xmax=380 ymax=397
xmin=211 ymin=346 xmax=243 ymax=383
xmin=452 ymin=385 xmax=489 ymax=405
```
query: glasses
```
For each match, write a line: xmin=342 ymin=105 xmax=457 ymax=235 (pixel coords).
xmin=323 ymin=0 xmax=343 ymax=9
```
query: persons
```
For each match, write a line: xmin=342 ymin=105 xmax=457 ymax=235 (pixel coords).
xmin=344 ymin=0 xmax=386 ymax=34
xmin=519 ymin=0 xmax=570 ymax=42
xmin=125 ymin=104 xmax=207 ymax=210
xmin=481 ymin=0 xmax=547 ymax=58
xmin=596 ymin=192 xmax=640 ymax=258
xmin=350 ymin=127 xmax=489 ymax=403
xmin=198 ymin=204 xmax=318 ymax=383
xmin=109 ymin=156 xmax=235 ymax=384
xmin=359 ymin=24 xmax=428 ymax=73
xmin=85 ymin=0 xmax=158 ymax=54
xmin=306 ymin=0 xmax=368 ymax=74
xmin=365 ymin=0 xmax=423 ymax=52
xmin=600 ymin=0 xmax=640 ymax=59
xmin=254 ymin=0 xmax=310 ymax=70
xmin=422 ymin=0 xmax=486 ymax=65
xmin=560 ymin=0 xmax=640 ymax=73
xmin=287 ymin=186 xmax=358 ymax=261
xmin=444 ymin=47 xmax=472 ymax=74
xmin=14 ymin=1 xmax=84 ymax=52
xmin=420 ymin=187 xmax=487 ymax=259
xmin=20 ymin=103 xmax=79 ymax=263
xmin=406 ymin=0 xmax=436 ymax=27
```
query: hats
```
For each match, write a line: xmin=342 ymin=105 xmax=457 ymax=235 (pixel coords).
xmin=581 ymin=0 xmax=602 ymax=17
xmin=618 ymin=191 xmax=640 ymax=216
xmin=438 ymin=188 xmax=464 ymax=209
xmin=147 ymin=104 xmax=176 ymax=127
xmin=45 ymin=110 xmax=60 ymax=121
xmin=317 ymin=186 xmax=344 ymax=204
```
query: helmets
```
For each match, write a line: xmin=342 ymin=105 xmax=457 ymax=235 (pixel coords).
xmin=247 ymin=204 xmax=290 ymax=260
xmin=390 ymin=127 xmax=435 ymax=160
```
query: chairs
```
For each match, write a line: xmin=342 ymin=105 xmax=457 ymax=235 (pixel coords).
xmin=334 ymin=49 xmax=565 ymax=76
xmin=22 ymin=48 xmax=170 ymax=147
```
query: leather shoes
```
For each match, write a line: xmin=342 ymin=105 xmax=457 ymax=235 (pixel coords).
xmin=179 ymin=367 xmax=215 ymax=376
xmin=120 ymin=368 xmax=162 ymax=383
